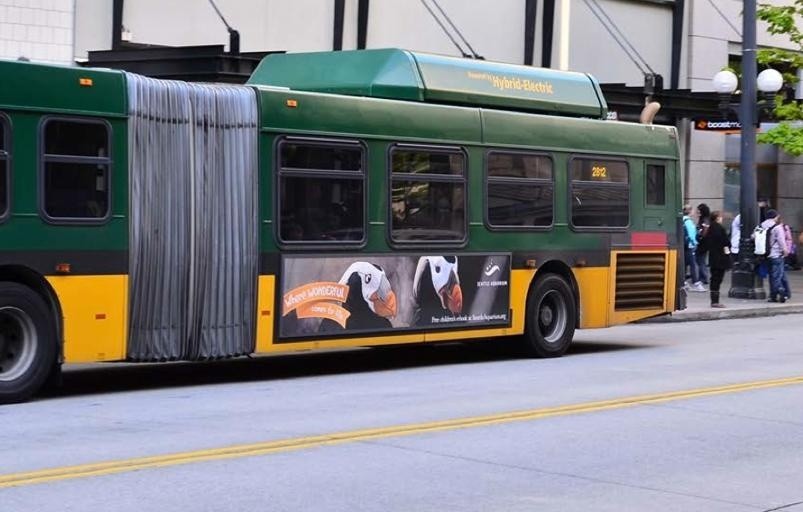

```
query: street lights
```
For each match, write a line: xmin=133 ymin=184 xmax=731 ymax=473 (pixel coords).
xmin=712 ymin=68 xmax=783 ymax=299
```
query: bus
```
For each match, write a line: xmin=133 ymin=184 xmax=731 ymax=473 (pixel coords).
xmin=0 ymin=47 xmax=687 ymax=405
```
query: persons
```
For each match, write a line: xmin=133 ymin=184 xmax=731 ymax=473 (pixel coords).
xmin=680 ymin=204 xmax=707 ymax=292
xmin=706 ymin=210 xmax=731 ymax=308
xmin=730 ymin=212 xmax=742 ymax=259
xmin=695 ymin=203 xmax=710 ymax=285
xmin=749 ymin=209 xmax=788 ymax=304
xmin=776 ymin=211 xmax=793 ymax=300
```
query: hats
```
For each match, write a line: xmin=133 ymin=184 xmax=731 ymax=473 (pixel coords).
xmin=766 ymin=208 xmax=778 ymax=218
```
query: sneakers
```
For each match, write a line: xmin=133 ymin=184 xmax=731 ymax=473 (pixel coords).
xmin=694 ymin=281 xmax=708 ymax=292
xmin=684 ymin=282 xmax=692 ymax=292
xmin=768 ymin=287 xmax=786 ymax=303
xmin=711 ymin=302 xmax=725 ymax=308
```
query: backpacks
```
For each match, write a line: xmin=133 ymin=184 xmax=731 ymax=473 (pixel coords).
xmin=683 ymin=218 xmax=690 ymax=254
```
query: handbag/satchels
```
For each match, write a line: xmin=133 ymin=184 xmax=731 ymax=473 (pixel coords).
xmin=753 ymin=223 xmax=782 ymax=256
